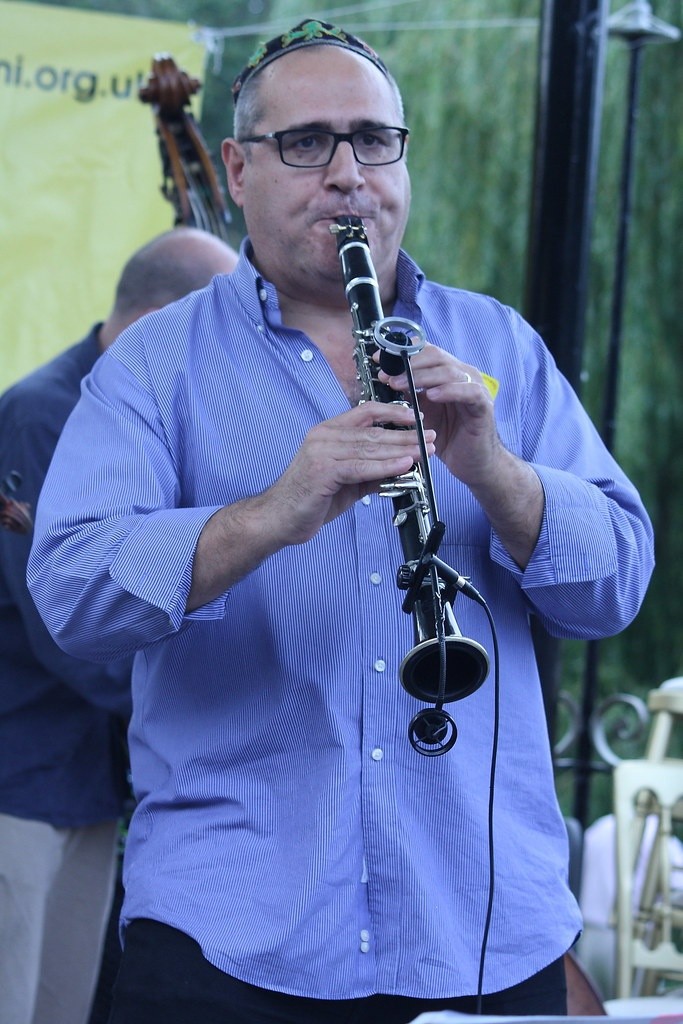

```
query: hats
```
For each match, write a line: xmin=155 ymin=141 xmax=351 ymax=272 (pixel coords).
xmin=230 ymin=18 xmax=404 ymax=124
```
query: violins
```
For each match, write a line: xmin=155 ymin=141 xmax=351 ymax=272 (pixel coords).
xmin=118 ymin=43 xmax=237 ymax=246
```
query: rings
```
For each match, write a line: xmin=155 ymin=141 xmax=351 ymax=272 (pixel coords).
xmin=465 ymin=372 xmax=471 ymax=382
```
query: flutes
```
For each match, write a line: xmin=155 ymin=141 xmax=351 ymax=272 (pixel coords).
xmin=332 ymin=217 xmax=488 ymax=703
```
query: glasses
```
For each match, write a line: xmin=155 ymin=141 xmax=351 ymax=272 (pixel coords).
xmin=240 ymin=128 xmax=410 ymax=168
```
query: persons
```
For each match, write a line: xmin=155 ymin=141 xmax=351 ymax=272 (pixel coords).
xmin=25 ymin=15 xmax=655 ymax=1024
xmin=0 ymin=229 xmax=244 ymax=1022
